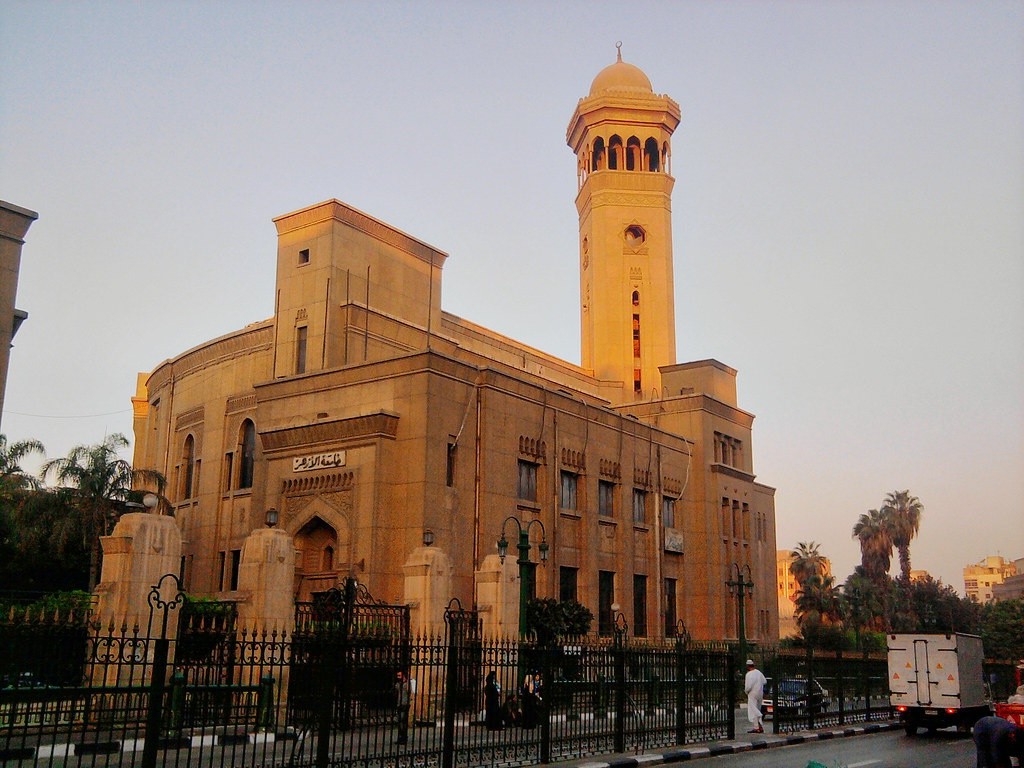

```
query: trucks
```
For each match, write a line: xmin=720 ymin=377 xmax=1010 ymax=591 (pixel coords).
xmin=995 ymin=660 xmax=1024 ymax=727
xmin=887 ymin=632 xmax=995 ymax=738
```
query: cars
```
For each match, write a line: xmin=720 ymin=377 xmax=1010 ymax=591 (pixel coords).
xmin=760 ymin=679 xmax=830 ymax=720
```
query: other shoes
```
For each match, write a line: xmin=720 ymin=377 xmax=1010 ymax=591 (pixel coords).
xmin=394 ymin=740 xmax=407 ymax=745
xmin=747 ymin=729 xmax=764 ymax=733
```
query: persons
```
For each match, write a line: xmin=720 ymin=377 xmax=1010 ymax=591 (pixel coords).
xmin=972 ymin=717 xmax=1024 ymax=768
xmin=1008 ymin=685 xmax=1024 ymax=723
xmin=484 ymin=671 xmax=543 ymax=731
xmin=745 ymin=660 xmax=767 ymax=733
xmin=395 ymin=670 xmax=418 ymax=746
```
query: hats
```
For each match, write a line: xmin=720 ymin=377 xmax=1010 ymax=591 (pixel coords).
xmin=746 ymin=659 xmax=753 ymax=664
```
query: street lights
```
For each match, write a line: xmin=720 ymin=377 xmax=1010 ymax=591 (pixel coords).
xmin=497 ymin=516 xmax=551 ymax=692
xmin=726 ymin=562 xmax=754 ymax=679
